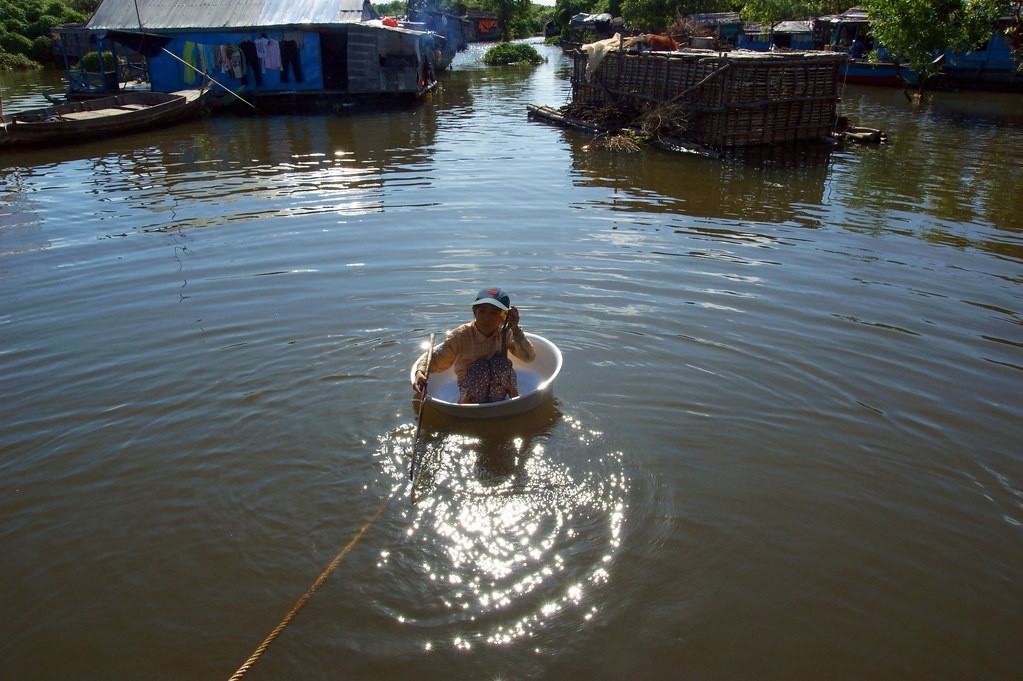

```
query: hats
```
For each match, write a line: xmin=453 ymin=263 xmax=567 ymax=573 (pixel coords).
xmin=470 ymin=286 xmax=510 ymax=311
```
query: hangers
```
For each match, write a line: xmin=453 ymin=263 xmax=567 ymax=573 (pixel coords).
xmin=257 ymin=34 xmax=262 ymax=39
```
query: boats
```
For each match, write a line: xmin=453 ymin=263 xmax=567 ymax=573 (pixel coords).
xmin=0 ymin=74 xmax=212 ymax=148
xmin=558 ymin=3 xmax=1023 ymax=96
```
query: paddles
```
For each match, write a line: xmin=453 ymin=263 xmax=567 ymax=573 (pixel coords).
xmin=407 ymin=330 xmax=437 ymax=482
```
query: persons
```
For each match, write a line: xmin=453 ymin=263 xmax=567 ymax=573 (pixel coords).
xmin=851 ymin=35 xmax=865 ymax=58
xmin=864 ymin=30 xmax=873 ymax=47
xmin=413 ymin=287 xmax=536 ymax=404
